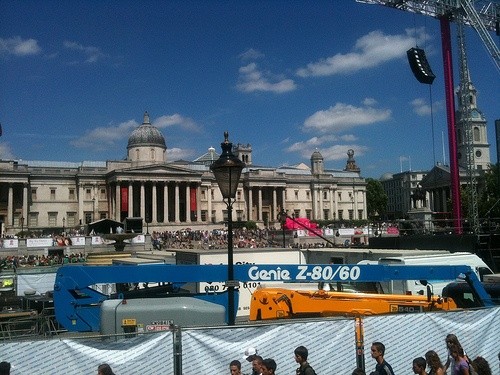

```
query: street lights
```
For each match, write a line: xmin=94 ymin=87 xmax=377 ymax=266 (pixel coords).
xmin=280 ymin=209 xmax=287 ymax=248
xmin=210 ymin=131 xmax=246 ymax=327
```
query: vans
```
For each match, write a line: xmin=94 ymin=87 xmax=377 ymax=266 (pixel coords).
xmin=376 ymin=253 xmax=495 ymax=301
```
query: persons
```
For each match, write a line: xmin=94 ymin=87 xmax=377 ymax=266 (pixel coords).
xmin=412 ymin=357 xmax=428 ymax=375
xmin=0 ymin=252 xmax=87 ymax=269
xmin=151 ymin=223 xmax=363 ymax=251
xmin=230 ymin=360 xmax=246 ymax=375
xmin=294 ymin=346 xmax=317 ymax=375
xmin=370 ymin=342 xmax=395 ymax=375
xmin=261 ymin=359 xmax=277 ymax=375
xmin=444 ymin=334 xmax=493 ymax=375
xmin=252 ymin=356 xmax=263 ymax=375
xmin=425 ymin=350 xmax=446 ymax=375
xmin=97 ymin=364 xmax=115 ymax=375
